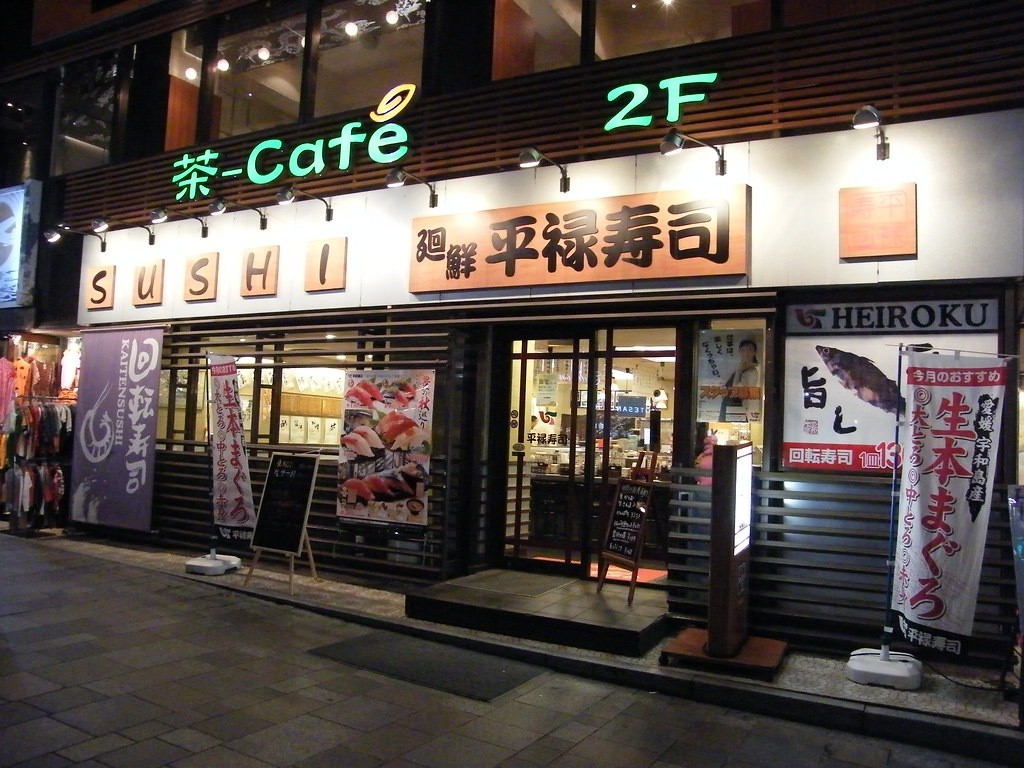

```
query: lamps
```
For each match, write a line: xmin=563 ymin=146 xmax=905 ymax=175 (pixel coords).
xmin=385 ymin=166 xmax=438 ymax=208
xmin=208 ymin=197 xmax=266 ymax=231
xmin=519 ymin=146 xmax=570 ymax=194
xmin=301 ymin=36 xmax=305 ymax=48
xmin=385 ymin=10 xmax=398 ymax=24
xmin=149 ymin=206 xmax=208 ymax=238
xmin=345 ymin=22 xmax=358 ymax=36
xmin=852 ymin=106 xmax=890 ymax=161
xmin=257 ymin=47 xmax=271 ymax=60
xmin=277 ymin=184 xmax=333 ymax=222
xmin=218 ymin=59 xmax=229 ymax=72
xmin=44 ymin=225 xmax=106 ymax=252
xmin=185 ymin=67 xmax=197 ymax=80
xmin=91 ymin=216 xmax=155 ymax=245
xmin=659 ymin=127 xmax=727 ymax=176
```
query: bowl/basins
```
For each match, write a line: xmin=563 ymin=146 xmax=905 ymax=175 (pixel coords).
xmin=407 ymin=498 xmax=425 ymax=515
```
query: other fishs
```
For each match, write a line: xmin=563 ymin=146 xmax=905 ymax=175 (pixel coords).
xmin=816 ymin=345 xmax=906 ymax=416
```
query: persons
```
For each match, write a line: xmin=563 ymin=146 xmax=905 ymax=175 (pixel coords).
xmin=529 ymin=415 xmax=539 ymax=431
xmin=719 ymin=339 xmax=762 ymax=422
xmin=692 ymin=435 xmax=719 ymax=485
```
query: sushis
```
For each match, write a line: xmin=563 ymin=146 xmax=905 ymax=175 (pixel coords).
xmin=341 ymin=380 xmax=426 ymax=502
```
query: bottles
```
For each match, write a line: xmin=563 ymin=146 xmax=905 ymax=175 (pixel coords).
xmin=553 ymin=447 xmax=672 ymax=476
